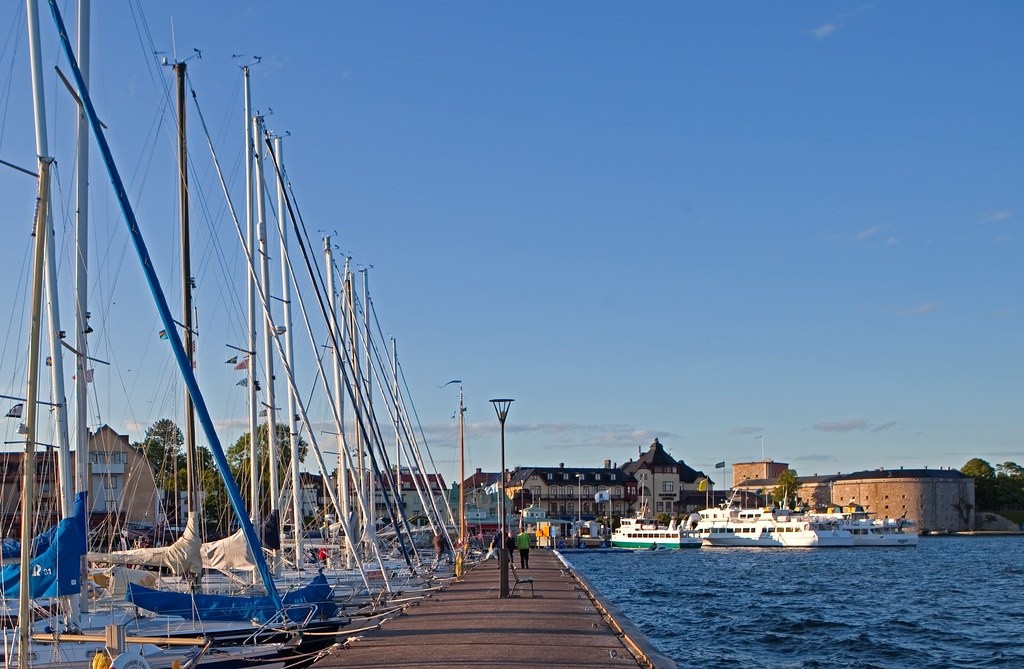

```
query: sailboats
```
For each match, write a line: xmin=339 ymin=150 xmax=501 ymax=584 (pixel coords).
xmin=1 ymin=2 xmax=518 ymax=669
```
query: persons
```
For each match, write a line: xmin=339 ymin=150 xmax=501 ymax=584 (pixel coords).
xmin=482 ymin=527 xmax=505 ymax=569
xmin=516 ymin=526 xmax=532 ymax=570
xmin=505 ymin=530 xmax=516 ymax=569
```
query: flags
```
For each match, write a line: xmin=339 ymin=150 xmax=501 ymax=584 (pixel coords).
xmin=594 ymin=489 xmax=610 ymax=504
xmin=696 ymin=478 xmax=708 ymax=492
xmin=636 ymin=477 xmax=644 ymax=491
xmin=715 ymin=462 xmax=725 ymax=469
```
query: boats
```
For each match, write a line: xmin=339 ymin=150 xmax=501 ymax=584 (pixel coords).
xmin=690 ymin=487 xmax=855 ymax=548
xmin=605 ymin=499 xmax=705 ymax=550
xmin=810 ymin=501 xmax=920 ymax=547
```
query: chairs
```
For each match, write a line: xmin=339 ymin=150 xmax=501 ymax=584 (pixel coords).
xmin=510 ymin=564 xmax=535 ymax=598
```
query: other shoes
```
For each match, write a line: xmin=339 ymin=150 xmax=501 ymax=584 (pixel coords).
xmin=510 ymin=564 xmax=517 ymax=569
xmin=522 ymin=567 xmax=524 ymax=569
xmin=526 ymin=567 xmax=528 ymax=569
xmin=497 ymin=566 xmax=499 ymax=569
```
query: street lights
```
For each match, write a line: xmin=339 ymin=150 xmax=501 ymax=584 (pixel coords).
xmin=519 ymin=478 xmax=526 ymax=528
xmin=575 ymin=473 xmax=582 ymax=537
xmin=487 ymin=397 xmax=516 ymax=599
xmin=745 ymin=477 xmax=750 ymax=508
